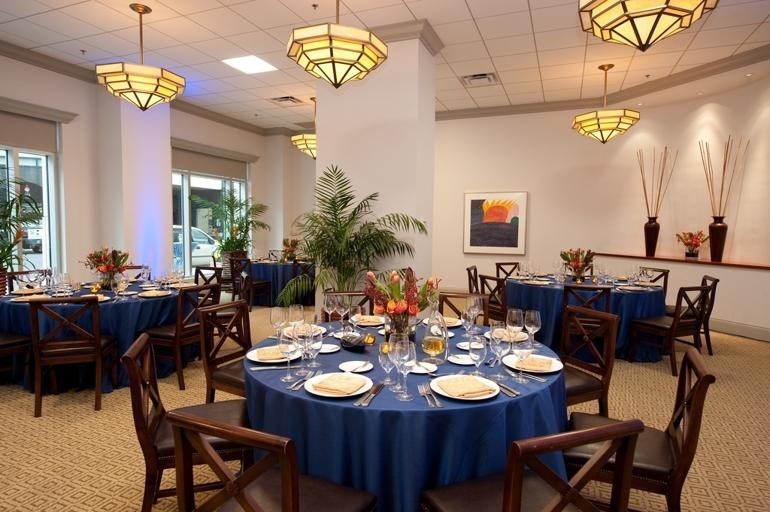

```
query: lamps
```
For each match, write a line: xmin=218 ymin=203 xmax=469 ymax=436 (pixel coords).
xmin=573 ymin=0 xmax=717 ymax=53
xmin=289 ymin=97 xmax=316 ymax=160
xmin=285 ymin=0 xmax=389 ymax=90
xmin=97 ymin=3 xmax=186 ymax=112
xmin=571 ymin=64 xmax=640 ymax=146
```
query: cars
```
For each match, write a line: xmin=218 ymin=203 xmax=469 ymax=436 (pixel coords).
xmin=173 ymin=225 xmax=222 ymax=267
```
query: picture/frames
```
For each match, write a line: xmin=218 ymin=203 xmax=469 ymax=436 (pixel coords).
xmin=462 ymin=191 xmax=528 ymax=256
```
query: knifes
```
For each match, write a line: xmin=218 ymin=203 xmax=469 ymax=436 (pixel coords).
xmin=249 ymin=365 xmax=303 ymax=372
xmin=353 ymin=384 xmax=386 ymax=406
xmin=473 ymin=371 xmax=520 ymax=398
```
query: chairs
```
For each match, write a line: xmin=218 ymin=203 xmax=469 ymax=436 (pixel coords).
xmin=564 ymin=344 xmax=717 ymax=512
xmin=3 ymin=265 xmax=254 ymax=391
xmin=120 ymin=333 xmax=253 ymax=512
xmin=210 ymin=250 xmax=322 ymax=310
xmin=323 ymin=291 xmax=373 ymax=323
xmin=30 ymin=295 xmax=117 ymax=418
xmin=461 ymin=255 xmax=721 ymax=375
xmin=439 ymin=291 xmax=490 ymax=328
xmin=425 ymin=416 xmax=644 ymax=510
xmin=559 ymin=303 xmax=618 ymax=416
xmin=166 ymin=411 xmax=375 ymax=512
xmin=199 ymin=300 xmax=254 ymax=404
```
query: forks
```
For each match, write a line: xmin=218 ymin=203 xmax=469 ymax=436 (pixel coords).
xmin=418 ymin=383 xmax=443 ymax=409
xmin=285 ymin=370 xmax=324 ymax=392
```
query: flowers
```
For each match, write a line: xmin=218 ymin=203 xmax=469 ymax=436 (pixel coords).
xmin=559 ymin=250 xmax=596 ymax=286
xmin=360 ymin=267 xmax=442 ymax=336
xmin=675 ymin=229 xmax=710 ymax=248
xmin=281 ymin=239 xmax=298 ymax=252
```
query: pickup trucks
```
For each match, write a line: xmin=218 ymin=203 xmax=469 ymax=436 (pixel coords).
xmin=22 ymin=226 xmax=43 ymax=252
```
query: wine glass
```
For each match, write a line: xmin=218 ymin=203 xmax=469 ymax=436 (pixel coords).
xmin=397 ymin=340 xmax=414 ymax=401
xmin=510 ymin=262 xmax=657 ymax=293
xmin=270 ymin=294 xmax=543 ymax=384
xmin=15 ymin=265 xmax=186 ymax=303
xmin=388 ymin=333 xmax=409 ymax=392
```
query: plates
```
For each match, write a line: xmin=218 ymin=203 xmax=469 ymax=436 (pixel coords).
xmin=9 ymin=288 xmax=51 ymax=303
xmin=428 ymin=373 xmax=500 ymax=401
xmin=504 ymin=353 xmax=564 ymax=375
xmin=246 ymin=344 xmax=301 ymax=363
xmin=304 ymin=373 xmax=373 ymax=397
xmin=169 ymin=282 xmax=198 ymax=289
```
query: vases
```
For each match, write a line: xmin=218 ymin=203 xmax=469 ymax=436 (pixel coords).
xmin=382 ymin=312 xmax=409 ymax=343
xmin=684 ymin=246 xmax=699 ymax=256
xmin=575 ymin=269 xmax=584 ymax=283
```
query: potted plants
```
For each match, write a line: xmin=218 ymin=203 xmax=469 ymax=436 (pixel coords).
xmin=629 ymin=143 xmax=680 ymax=256
xmin=190 ymin=186 xmax=270 ymax=275
xmin=697 ymin=133 xmax=751 ymax=262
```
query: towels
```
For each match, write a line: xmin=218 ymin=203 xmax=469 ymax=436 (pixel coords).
xmin=437 ymin=374 xmax=496 ymax=399
xmin=286 ymin=325 xmax=321 ymax=335
xmin=516 ymin=358 xmax=552 ymax=372
xmin=256 ymin=347 xmax=289 ymax=361
xmin=501 ymin=330 xmax=525 ymax=342
xmin=310 ymin=374 xmax=365 ymax=395
xmin=448 ymin=318 xmax=458 ymax=326
xmin=360 ymin=316 xmax=383 ymax=324
